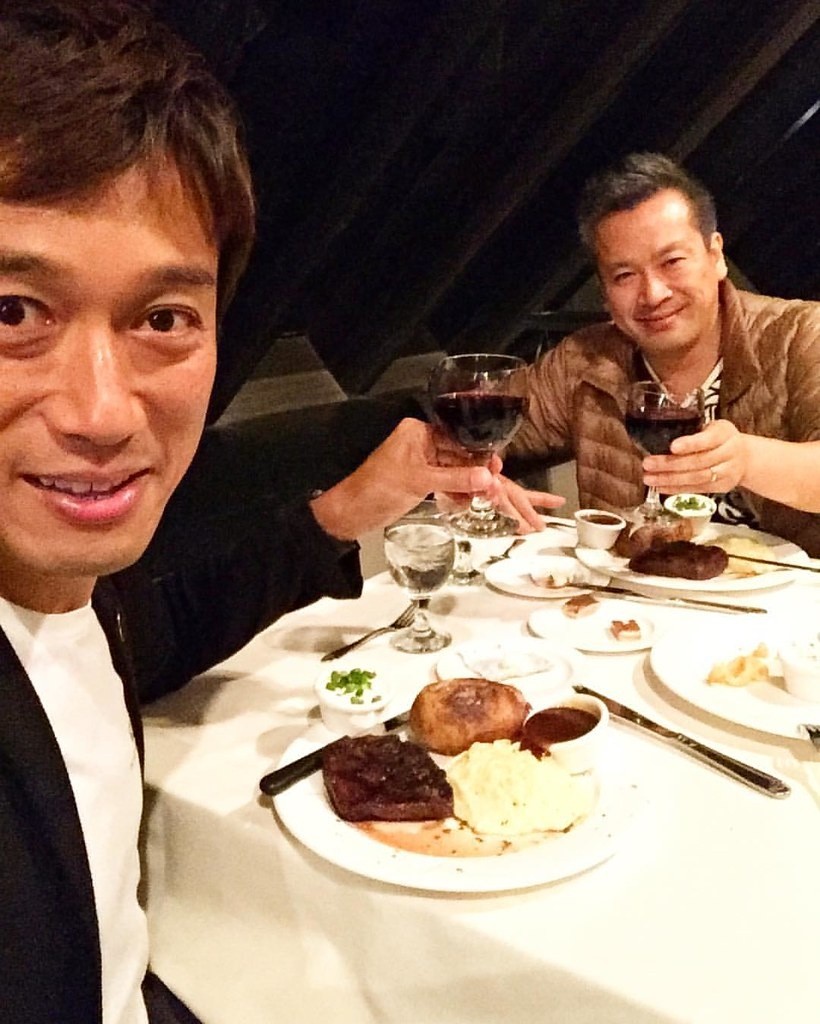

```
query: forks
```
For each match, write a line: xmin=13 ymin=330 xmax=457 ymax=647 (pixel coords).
xmin=320 ymin=603 xmax=420 ymax=661
xmin=801 ymin=724 xmax=820 ymax=753
xmin=489 ymin=536 xmax=526 ymax=560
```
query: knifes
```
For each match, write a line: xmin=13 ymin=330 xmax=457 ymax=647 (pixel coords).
xmin=574 ymin=685 xmax=791 ymax=799
xmin=260 ymin=710 xmax=410 ymax=796
xmin=565 ymin=582 xmax=767 ymax=613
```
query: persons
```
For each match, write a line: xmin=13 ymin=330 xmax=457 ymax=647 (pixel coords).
xmin=0 ymin=18 xmax=502 ymax=1024
xmin=442 ymin=151 xmax=820 ymax=560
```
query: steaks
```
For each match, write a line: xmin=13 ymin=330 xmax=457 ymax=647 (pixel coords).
xmin=324 ymin=734 xmax=453 ymax=821
xmin=628 ymin=540 xmax=730 ymax=581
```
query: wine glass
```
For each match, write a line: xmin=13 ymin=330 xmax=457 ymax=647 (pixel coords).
xmin=620 ymin=380 xmax=704 ymax=521
xmin=427 ymin=354 xmax=531 ymax=537
xmin=384 ymin=519 xmax=455 ymax=653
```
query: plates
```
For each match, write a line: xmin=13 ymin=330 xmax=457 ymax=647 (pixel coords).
xmin=484 ymin=555 xmax=611 ymax=598
xmin=574 ymin=522 xmax=809 ymax=590
xmin=649 ymin=613 xmax=820 ymax=740
xmin=271 ymin=721 xmax=640 ymax=893
xmin=527 ymin=599 xmax=652 ymax=651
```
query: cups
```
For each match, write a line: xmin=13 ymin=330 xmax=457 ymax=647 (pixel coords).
xmin=315 ymin=662 xmax=391 ymax=734
xmin=574 ymin=508 xmax=625 ymax=550
xmin=663 ymin=492 xmax=716 ymax=536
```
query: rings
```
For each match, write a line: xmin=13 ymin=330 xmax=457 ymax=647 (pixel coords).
xmin=710 ymin=467 xmax=716 ymax=481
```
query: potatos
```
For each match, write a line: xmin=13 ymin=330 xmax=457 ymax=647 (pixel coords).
xmin=404 ymin=677 xmax=530 ymax=756
xmin=615 ymin=510 xmax=692 ymax=558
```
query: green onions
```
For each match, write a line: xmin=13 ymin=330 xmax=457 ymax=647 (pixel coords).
xmin=673 ymin=495 xmax=709 ymax=510
xmin=327 ymin=667 xmax=383 ymax=704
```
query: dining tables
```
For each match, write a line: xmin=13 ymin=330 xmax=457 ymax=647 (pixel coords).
xmin=120 ymin=502 xmax=820 ymax=1024
